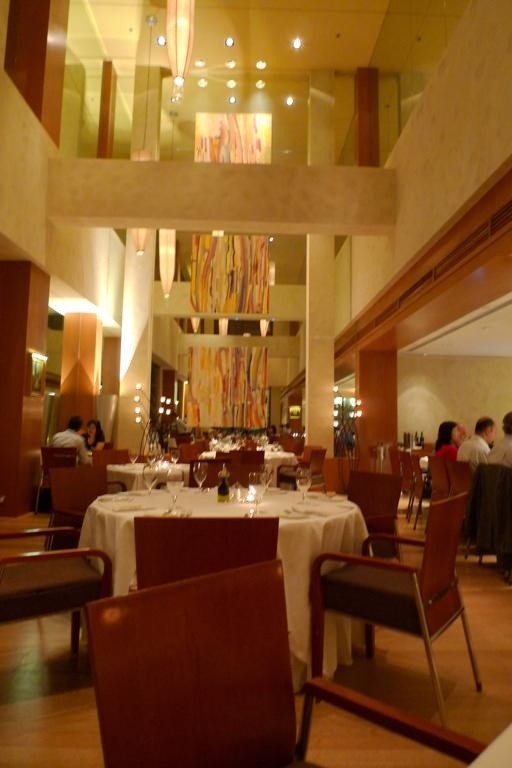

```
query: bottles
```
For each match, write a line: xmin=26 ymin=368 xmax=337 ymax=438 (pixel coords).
xmin=414 ymin=432 xmax=418 ymax=445
xmin=420 ymin=432 xmax=424 ymax=447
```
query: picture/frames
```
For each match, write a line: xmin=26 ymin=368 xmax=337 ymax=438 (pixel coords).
xmin=28 ymin=353 xmax=48 ymax=399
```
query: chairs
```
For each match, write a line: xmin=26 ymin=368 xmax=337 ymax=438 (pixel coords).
xmin=0 ymin=528 xmax=111 ymax=688
xmin=311 ymin=491 xmax=483 ymax=731
xmin=44 ymin=467 xmax=107 ymax=551
xmin=388 ymin=443 xmax=512 ymax=585
xmin=34 ymin=433 xmax=326 ymax=514
xmin=128 ymin=517 xmax=280 ymax=593
xmin=83 ymin=556 xmax=488 ymax=768
xmin=347 ymin=472 xmax=406 ymax=567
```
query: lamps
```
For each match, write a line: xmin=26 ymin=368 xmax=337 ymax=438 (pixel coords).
xmin=133 ymin=384 xmax=180 ymax=463
xmin=191 ymin=230 xmax=275 ymax=337
xmin=130 ymin=15 xmax=159 ymax=256
xmin=165 ymin=0 xmax=195 ymax=87
xmin=332 ymin=386 xmax=362 ymax=496
xmin=159 ymin=111 xmax=178 ymax=299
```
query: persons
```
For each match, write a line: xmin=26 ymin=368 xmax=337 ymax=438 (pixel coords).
xmin=487 ymin=411 xmax=512 ymax=469
xmin=170 ymin=410 xmax=190 ymax=435
xmin=457 ymin=423 xmax=470 ymax=447
xmin=81 ymin=419 xmax=106 ymax=452
xmin=456 ymin=417 xmax=498 ymax=469
xmin=433 ymin=422 xmax=461 ymax=461
xmin=268 ymin=425 xmax=280 ymax=444
xmin=43 ymin=416 xmax=88 ymax=503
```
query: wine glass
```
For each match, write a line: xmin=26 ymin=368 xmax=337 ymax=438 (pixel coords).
xmin=143 ymin=469 xmax=157 ymax=495
xmin=156 ymin=450 xmax=164 ymax=467
xmin=146 ymin=452 xmax=156 ymax=465
xmin=164 ymin=468 xmax=185 ymax=513
xmin=128 ymin=448 xmax=138 ymax=464
xmin=193 ymin=461 xmax=209 ymax=488
xmin=170 ymin=449 xmax=180 ymax=464
xmin=248 ymin=472 xmax=267 ymax=514
xmin=295 ymin=468 xmax=311 ymax=501
xmin=260 ymin=464 xmax=274 ymax=490
